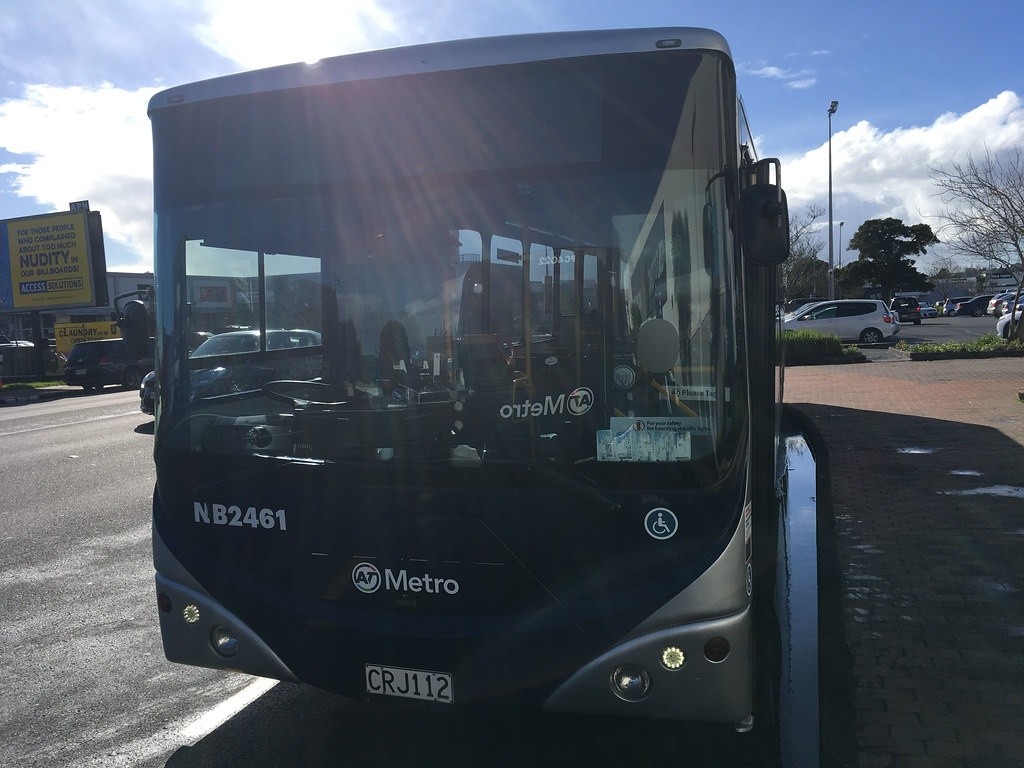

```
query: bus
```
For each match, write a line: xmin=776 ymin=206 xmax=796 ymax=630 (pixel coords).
xmin=114 ymin=25 xmax=789 ymax=730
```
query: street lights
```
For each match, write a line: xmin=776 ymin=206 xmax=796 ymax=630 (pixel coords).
xmin=827 ymin=100 xmax=839 ymax=299
xmin=839 ymin=221 xmax=844 ymax=269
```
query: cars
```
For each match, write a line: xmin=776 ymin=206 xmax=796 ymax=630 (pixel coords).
xmin=935 ymin=287 xmax=1024 ymax=339
xmin=918 ymin=302 xmax=937 ymax=319
xmin=138 ymin=328 xmax=323 ymax=417
xmin=787 ymin=297 xmax=839 ymax=320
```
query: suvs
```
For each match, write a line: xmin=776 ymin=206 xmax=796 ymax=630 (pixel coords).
xmin=783 ymin=299 xmax=900 ymax=344
xmin=62 ymin=337 xmax=158 ymax=392
xmin=888 ymin=296 xmax=921 ymax=325
